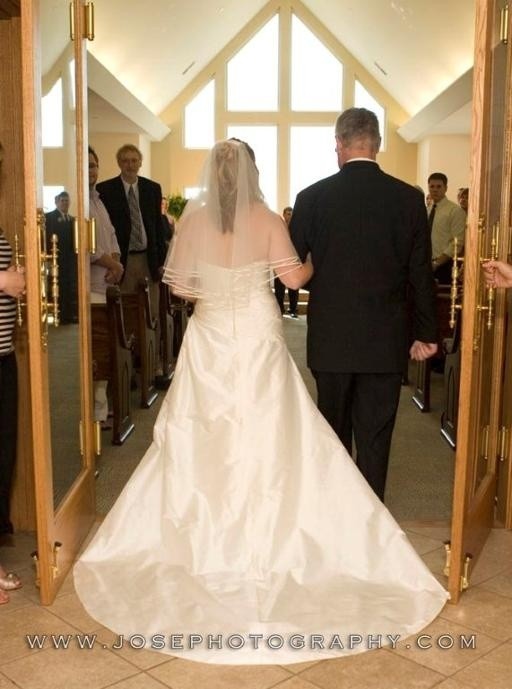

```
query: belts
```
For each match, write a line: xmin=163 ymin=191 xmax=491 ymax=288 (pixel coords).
xmin=128 ymin=250 xmax=148 ymax=255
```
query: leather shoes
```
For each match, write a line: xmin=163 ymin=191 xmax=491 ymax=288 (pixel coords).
xmin=101 ymin=410 xmax=113 ymax=430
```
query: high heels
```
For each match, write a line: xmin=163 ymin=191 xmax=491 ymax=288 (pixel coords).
xmin=0 ymin=570 xmax=24 ymax=605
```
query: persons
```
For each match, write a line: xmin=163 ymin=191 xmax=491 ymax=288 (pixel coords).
xmin=159 ymin=136 xmax=314 ymax=563
xmin=0 ymin=143 xmax=28 ymax=606
xmin=44 ymin=143 xmax=470 ymax=433
xmin=478 ymin=258 xmax=512 ymax=292
xmin=287 ymin=107 xmax=443 ymax=505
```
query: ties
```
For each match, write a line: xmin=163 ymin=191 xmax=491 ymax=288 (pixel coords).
xmin=429 ymin=204 xmax=437 ymax=231
xmin=128 ymin=184 xmax=143 ymax=250
xmin=64 ymin=214 xmax=68 ymax=222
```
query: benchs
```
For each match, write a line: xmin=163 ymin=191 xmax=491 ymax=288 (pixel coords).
xmin=89 ymin=268 xmax=195 ymax=445
xmin=400 ymin=283 xmax=461 ymax=451
